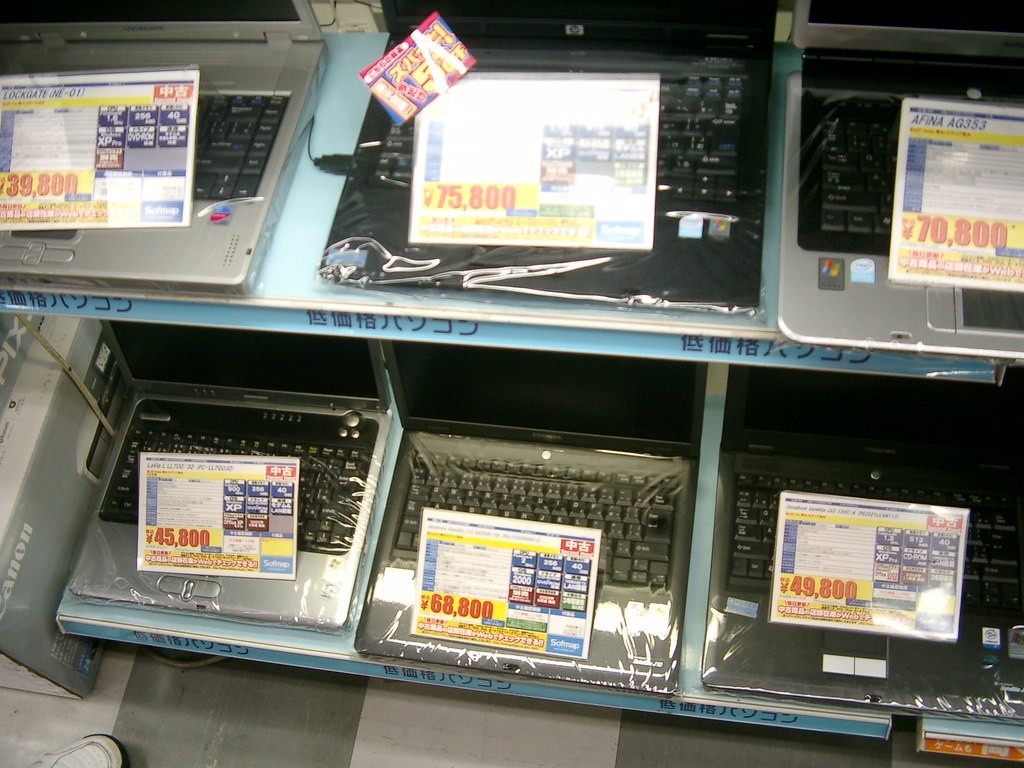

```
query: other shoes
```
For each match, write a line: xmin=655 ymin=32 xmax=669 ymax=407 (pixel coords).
xmin=25 ymin=732 xmax=128 ymax=768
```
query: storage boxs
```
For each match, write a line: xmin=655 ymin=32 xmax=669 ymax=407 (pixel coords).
xmin=0 ymin=312 xmax=131 ymax=700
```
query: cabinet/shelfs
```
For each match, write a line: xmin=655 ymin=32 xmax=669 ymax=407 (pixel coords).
xmin=0 ymin=33 xmax=1024 ymax=768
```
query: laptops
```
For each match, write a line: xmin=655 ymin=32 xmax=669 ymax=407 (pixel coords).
xmin=776 ymin=0 xmax=1024 ymax=366
xmin=320 ymin=0 xmax=780 ymax=310
xmin=701 ymin=363 xmax=1024 ymax=726
xmin=354 ymin=342 xmax=706 ymax=697
xmin=70 ymin=318 xmax=392 ymax=633
xmin=0 ymin=0 xmax=329 ymax=292
